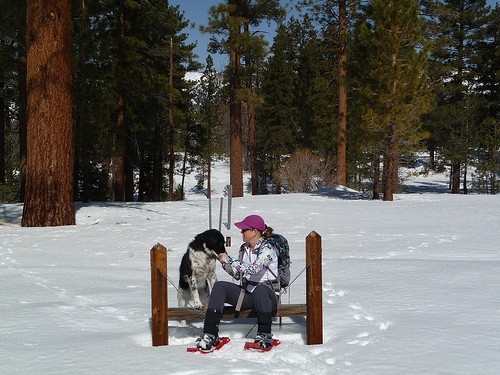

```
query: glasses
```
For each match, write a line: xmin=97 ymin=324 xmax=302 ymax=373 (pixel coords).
xmin=242 ymin=228 xmax=252 ymax=232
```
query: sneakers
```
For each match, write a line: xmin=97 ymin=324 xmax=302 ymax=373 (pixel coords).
xmin=197 ymin=333 xmax=219 ymax=350
xmin=254 ymin=332 xmax=274 ymax=347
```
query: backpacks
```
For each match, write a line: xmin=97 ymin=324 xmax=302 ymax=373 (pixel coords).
xmin=235 ymin=234 xmax=291 ymax=312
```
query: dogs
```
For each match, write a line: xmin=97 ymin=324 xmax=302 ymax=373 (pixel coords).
xmin=177 ymin=228 xmax=227 ymax=327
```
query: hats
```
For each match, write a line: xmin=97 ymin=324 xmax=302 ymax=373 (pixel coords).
xmin=234 ymin=214 xmax=266 ymax=231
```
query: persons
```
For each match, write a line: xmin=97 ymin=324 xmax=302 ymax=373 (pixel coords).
xmin=197 ymin=215 xmax=280 ymax=348
